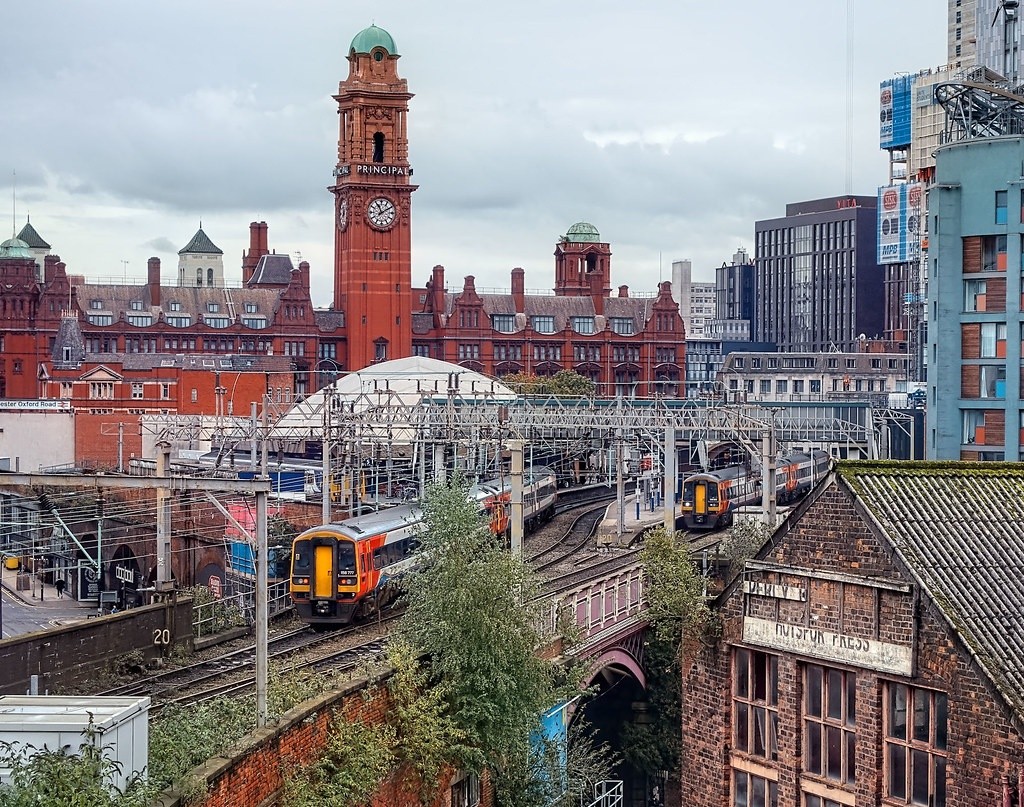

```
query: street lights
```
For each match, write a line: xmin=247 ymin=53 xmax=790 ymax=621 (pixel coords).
xmin=801 ymin=438 xmax=813 ymax=490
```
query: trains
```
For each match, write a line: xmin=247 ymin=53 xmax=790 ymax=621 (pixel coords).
xmin=681 ymin=450 xmax=831 ymax=530
xmin=289 ymin=465 xmax=557 ymax=632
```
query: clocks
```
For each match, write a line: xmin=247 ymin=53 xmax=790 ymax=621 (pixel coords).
xmin=368 ymin=198 xmax=395 ymax=228
xmin=340 ymin=200 xmax=347 ymax=228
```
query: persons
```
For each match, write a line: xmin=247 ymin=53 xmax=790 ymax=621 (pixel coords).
xmin=55 ymin=577 xmax=65 ymax=598
xmin=111 ymin=606 xmax=120 ymax=613
xmin=552 ymin=463 xmax=606 ymax=487
